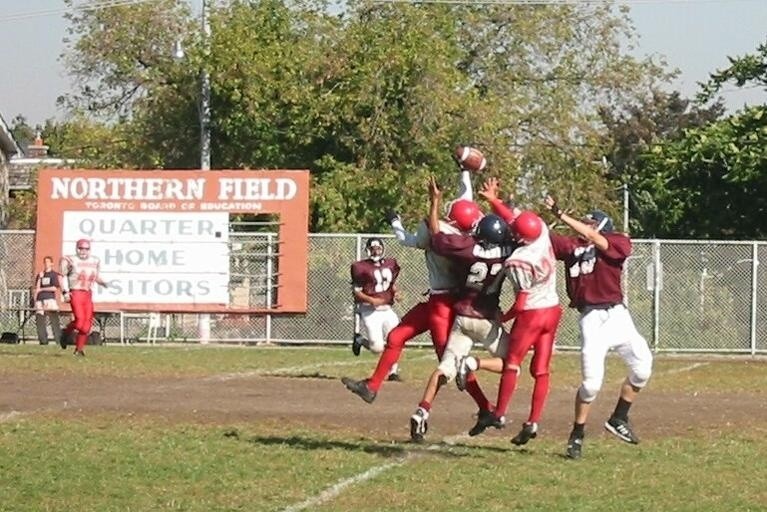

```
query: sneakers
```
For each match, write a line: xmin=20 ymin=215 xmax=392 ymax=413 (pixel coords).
xmin=341 ymin=377 xmax=377 ymax=405
xmin=479 ymin=414 xmax=506 ymax=430
xmin=387 ymin=373 xmax=401 ymax=382
xmin=408 ymin=413 xmax=426 ymax=444
xmin=511 ymin=422 xmax=538 ymax=446
xmin=455 ymin=355 xmax=472 ymax=392
xmin=352 ymin=334 xmax=361 ymax=356
xmin=60 ymin=329 xmax=67 ymax=349
xmin=604 ymin=417 xmax=639 ymax=445
xmin=469 ymin=411 xmax=495 ymax=436
xmin=73 ymin=349 xmax=85 ymax=357
xmin=567 ymin=431 xmax=585 ymax=459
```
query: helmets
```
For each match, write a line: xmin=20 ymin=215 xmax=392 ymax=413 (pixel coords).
xmin=511 ymin=212 xmax=542 ymax=245
xmin=443 ymin=200 xmax=479 ymax=232
xmin=76 ymin=239 xmax=90 ymax=259
xmin=580 ymin=211 xmax=613 ymax=236
xmin=366 ymin=237 xmax=386 ymax=263
xmin=476 ymin=214 xmax=507 ymax=247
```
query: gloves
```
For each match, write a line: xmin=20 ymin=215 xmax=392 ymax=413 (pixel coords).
xmin=497 ymin=191 xmax=515 ymax=212
xmin=451 ymin=155 xmax=470 ymax=172
xmin=548 ymin=201 xmax=563 ymax=218
xmin=380 ymin=205 xmax=399 ymax=226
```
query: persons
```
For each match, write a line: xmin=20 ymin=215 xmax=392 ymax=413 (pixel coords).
xmin=33 ymin=256 xmax=71 ymax=346
xmin=58 ymin=239 xmax=108 ymax=357
xmin=477 ymin=176 xmax=562 ymax=445
xmin=411 ymin=169 xmax=522 ymax=445
xmin=350 ymin=237 xmax=405 ymax=384
xmin=537 ymin=194 xmax=654 ymax=459
xmin=340 ymin=157 xmax=499 ymax=438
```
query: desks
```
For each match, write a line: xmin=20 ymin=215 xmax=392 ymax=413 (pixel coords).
xmin=6 ymin=307 xmax=120 ymax=347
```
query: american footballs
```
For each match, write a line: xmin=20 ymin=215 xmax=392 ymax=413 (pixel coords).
xmin=456 ymin=146 xmax=487 ymax=171
